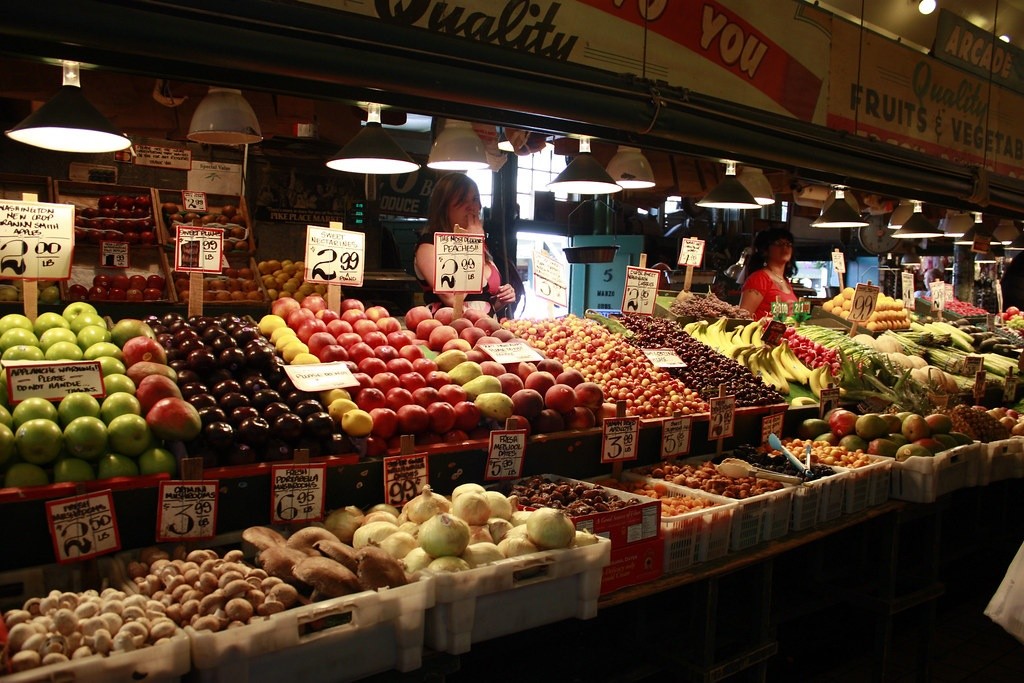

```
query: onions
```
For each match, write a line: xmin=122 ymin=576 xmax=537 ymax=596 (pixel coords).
xmin=324 ymin=485 xmax=600 ymax=580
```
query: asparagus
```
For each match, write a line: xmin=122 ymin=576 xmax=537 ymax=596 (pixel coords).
xmin=885 ymin=329 xmax=1004 ymax=393
xmin=794 ymin=326 xmax=878 ymax=362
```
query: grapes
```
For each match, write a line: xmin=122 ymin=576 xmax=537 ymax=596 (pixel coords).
xmin=1006 ymin=316 xmax=1024 ymax=338
xmin=669 ymin=292 xmax=751 ymax=319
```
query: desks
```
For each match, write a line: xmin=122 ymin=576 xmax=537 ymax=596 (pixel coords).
xmin=595 ymin=499 xmax=949 ymax=683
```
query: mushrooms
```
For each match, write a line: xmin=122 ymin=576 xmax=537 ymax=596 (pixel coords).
xmin=3 ymin=525 xmax=412 ymax=673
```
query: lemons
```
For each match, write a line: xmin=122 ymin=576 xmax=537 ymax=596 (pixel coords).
xmin=257 ymin=313 xmax=374 ymax=437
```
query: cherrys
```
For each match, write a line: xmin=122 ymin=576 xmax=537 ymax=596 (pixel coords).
xmin=501 ymin=314 xmax=713 ymax=420
xmin=618 ymin=315 xmax=787 ymax=407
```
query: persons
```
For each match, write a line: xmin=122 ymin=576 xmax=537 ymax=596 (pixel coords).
xmin=923 ymin=268 xmax=941 ymax=298
xmin=737 ymin=227 xmax=801 ymax=327
xmin=412 ymin=172 xmax=517 ymax=325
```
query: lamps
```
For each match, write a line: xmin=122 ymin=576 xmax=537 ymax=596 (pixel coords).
xmin=953 ymin=213 xmax=1001 ymax=245
xmin=185 ymin=87 xmax=264 ymax=146
xmin=992 ymin=219 xmax=1020 ymax=246
xmin=545 ymin=134 xmax=622 ymax=195
xmin=427 ymin=118 xmax=491 ymax=171
xmin=808 ymin=188 xmax=870 ymax=228
xmin=1003 ymin=223 xmax=1024 ymax=251
xmin=900 ymin=245 xmax=921 ymax=266
xmin=887 ymin=199 xmax=914 ymax=230
xmin=4 ymin=60 xmax=133 ymax=154
xmin=325 ymin=102 xmax=422 ymax=176
xmin=819 ymin=184 xmax=863 ymax=216
xmin=737 ymin=166 xmax=775 ymax=206
xmin=693 ymin=160 xmax=763 ymax=210
xmin=877 ymin=253 xmax=901 ymax=271
xmin=972 ymin=249 xmax=997 ymax=264
xmin=891 ymin=202 xmax=945 ymax=239
xmin=606 ymin=145 xmax=657 ymax=190
xmin=943 ymin=261 xmax=953 ymax=271
xmin=497 ymin=131 xmax=514 ymax=152
xmin=944 ymin=210 xmax=975 ymax=238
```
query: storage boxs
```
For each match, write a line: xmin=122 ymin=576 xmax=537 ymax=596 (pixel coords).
xmin=163 ymin=251 xmax=272 ymax=312
xmin=483 ymin=474 xmax=662 ymax=552
xmin=804 ymin=317 xmax=861 ymax=337
xmin=822 ymin=450 xmax=895 ymax=514
xmin=0 ymin=555 xmax=192 ymax=683
xmin=597 ymin=534 xmax=666 ymax=597
xmin=68 ymin=161 xmax=120 ymax=186
xmin=653 ymin=295 xmax=754 ymax=333
xmin=0 ymin=278 xmax=64 ymax=316
xmin=810 ymin=305 xmax=914 ymax=340
xmin=154 ymin=187 xmax=257 ymax=257
xmin=64 ymin=246 xmax=174 ymax=322
xmin=692 ymin=448 xmax=851 ymax=532
xmin=887 ymin=440 xmax=982 ymax=503
xmin=425 ymin=530 xmax=611 ymax=656
xmin=112 ymin=524 xmax=437 ymax=683
xmin=51 ymin=179 xmax=163 ymax=248
xmin=981 ymin=435 xmax=1024 ymax=486
xmin=582 ymin=470 xmax=739 ymax=574
xmin=915 ymin=296 xmax=988 ymax=325
xmin=0 ymin=172 xmax=54 ymax=204
xmin=631 ymin=455 xmax=798 ymax=553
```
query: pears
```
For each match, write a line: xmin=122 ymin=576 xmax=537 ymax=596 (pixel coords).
xmin=447 ymin=361 xmax=483 ymax=384
xmin=473 ymin=391 xmax=515 ymax=421
xmin=259 ymin=259 xmax=330 ymax=301
xmin=0 ymin=277 xmax=61 ymax=302
xmin=461 ymin=375 xmax=502 ymax=401
xmin=433 ymin=349 xmax=467 ymax=371
xmin=821 ymin=288 xmax=911 ymax=331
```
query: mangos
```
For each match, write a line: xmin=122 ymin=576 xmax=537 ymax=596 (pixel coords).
xmin=968 ymin=403 xmax=1024 ymax=437
xmin=798 ymin=407 xmax=973 ymax=462
xmin=111 ymin=318 xmax=202 ymax=439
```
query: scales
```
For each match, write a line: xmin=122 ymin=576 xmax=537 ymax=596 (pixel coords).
xmin=858 ymin=196 xmax=903 ymax=298
xmin=663 ymin=194 xmax=718 ymax=285
xmin=547 ymin=136 xmax=621 ymax=264
xmin=340 ymin=270 xmax=416 ymax=316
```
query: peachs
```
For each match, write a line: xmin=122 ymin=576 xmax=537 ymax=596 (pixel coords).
xmin=270 ymin=294 xmax=618 ymax=455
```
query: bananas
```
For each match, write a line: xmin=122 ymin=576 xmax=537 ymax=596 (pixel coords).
xmin=684 ymin=316 xmax=847 ymax=407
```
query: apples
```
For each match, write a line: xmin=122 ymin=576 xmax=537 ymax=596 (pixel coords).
xmin=67 ymin=195 xmax=265 ymax=301
xmin=1 ymin=301 xmax=176 ymax=490
xmin=994 ymin=307 xmax=1024 ymax=320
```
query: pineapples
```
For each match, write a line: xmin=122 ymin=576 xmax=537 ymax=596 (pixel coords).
xmin=831 ymin=348 xmax=1009 ymax=443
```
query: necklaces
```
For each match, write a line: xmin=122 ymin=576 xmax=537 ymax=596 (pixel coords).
xmin=769 ymin=267 xmax=785 ymax=284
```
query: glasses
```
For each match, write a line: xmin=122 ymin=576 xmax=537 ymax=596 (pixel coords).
xmin=771 ymin=240 xmax=792 ymax=247
